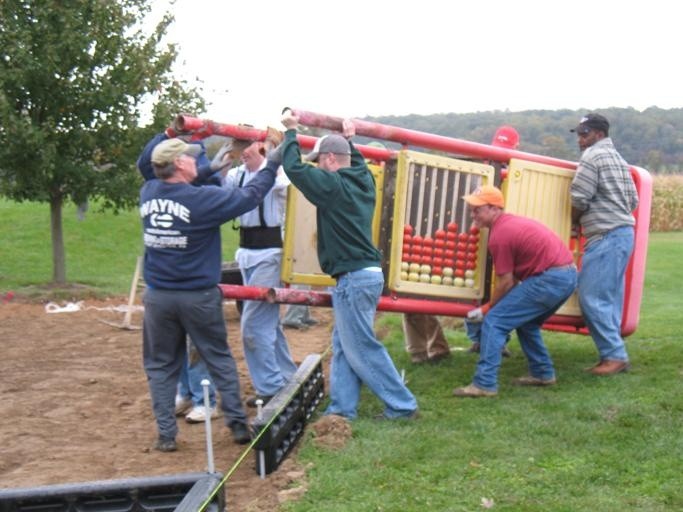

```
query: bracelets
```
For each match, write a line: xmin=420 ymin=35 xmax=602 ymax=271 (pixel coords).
xmin=481 ymin=304 xmax=491 ymax=314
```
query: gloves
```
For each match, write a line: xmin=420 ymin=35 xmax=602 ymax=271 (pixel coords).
xmin=165 ymin=113 xmax=283 ymax=170
xmin=466 ymin=304 xmax=491 ymax=324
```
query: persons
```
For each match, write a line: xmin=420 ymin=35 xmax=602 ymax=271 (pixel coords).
xmin=281 ymin=109 xmax=419 ymax=422
xmin=565 ymin=113 xmax=640 ymax=374
xmin=362 ymin=142 xmax=451 ymax=367
xmin=453 ymin=185 xmax=578 ymax=397
xmin=136 ymin=122 xmax=300 ymax=454
xmin=463 ymin=126 xmax=519 ymax=359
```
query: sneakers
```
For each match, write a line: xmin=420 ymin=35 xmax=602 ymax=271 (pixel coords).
xmin=155 ymin=392 xmax=271 ymax=450
xmin=454 ymin=386 xmax=496 ymax=396
xmin=517 ymin=376 xmax=556 ymax=386
xmin=584 ymin=359 xmax=630 ymax=376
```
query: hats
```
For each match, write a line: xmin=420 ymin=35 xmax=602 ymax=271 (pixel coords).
xmin=571 ymin=114 xmax=609 ymax=133
xmin=491 ymin=126 xmax=520 ymax=149
xmin=305 ymin=136 xmax=351 ymax=162
xmin=461 ymin=186 xmax=504 ymax=207
